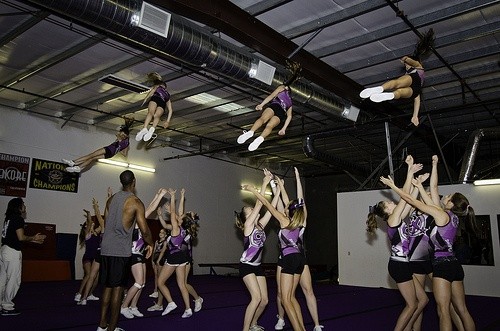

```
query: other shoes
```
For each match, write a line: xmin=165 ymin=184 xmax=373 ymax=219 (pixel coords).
xmin=147 ymin=304 xmax=163 ymax=311
xmin=149 ymin=292 xmax=159 ymax=298
xmin=128 ymin=306 xmax=143 ymax=317
xmin=360 ymin=86 xmax=395 ymax=103
xmin=314 ymin=325 xmax=323 ymax=331
xmin=61 ymin=159 xmax=75 ymax=166
xmin=135 ymin=128 xmax=148 ymax=141
xmin=87 ymin=294 xmax=99 ymax=300
xmin=237 ymin=130 xmax=253 ymax=144
xmin=249 ymin=325 xmax=264 ymax=331
xmin=143 ymin=127 xmax=155 ymax=141
xmin=121 ymin=305 xmax=134 ymax=318
xmin=77 ymin=299 xmax=86 ymax=305
xmin=181 ymin=308 xmax=192 ymax=317
xmin=2 ymin=308 xmax=21 ymax=316
xmin=248 ymin=136 xmax=264 ymax=151
xmin=97 ymin=325 xmax=108 ymax=331
xmin=75 ymin=292 xmax=81 ymax=301
xmin=114 ymin=328 xmax=124 ymax=331
xmin=275 ymin=318 xmax=285 ymax=329
xmin=66 ymin=166 xmax=80 ymax=173
xmin=194 ymin=296 xmax=203 ymax=312
xmin=162 ymin=302 xmax=177 ymax=316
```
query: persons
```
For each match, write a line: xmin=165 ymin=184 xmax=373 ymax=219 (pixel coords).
xmin=155 ymin=186 xmax=204 ymax=318
xmin=237 ymin=60 xmax=303 ymax=153
xmin=149 ymin=228 xmax=169 ymax=298
xmin=359 ymin=27 xmax=439 ymax=127
xmin=74 ymin=184 xmax=146 ymax=319
xmin=134 ymin=72 xmax=173 ymax=143
xmin=233 ymin=165 xmax=324 ymax=331
xmin=96 ymin=170 xmax=154 ymax=331
xmin=0 ymin=197 xmax=47 ymax=315
xmin=363 ymin=154 xmax=480 ymax=331
xmin=62 ymin=116 xmax=134 ymax=173
